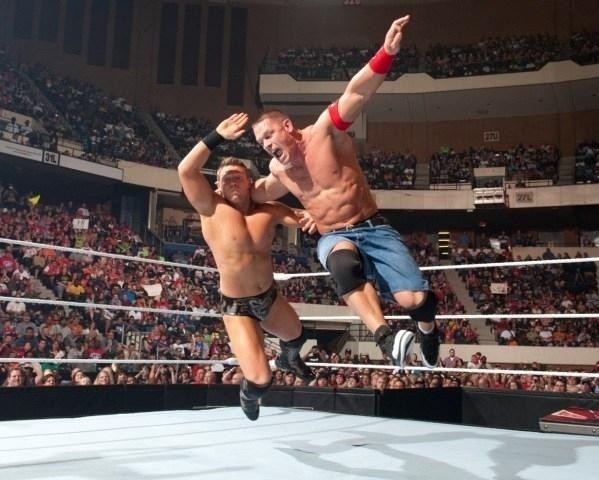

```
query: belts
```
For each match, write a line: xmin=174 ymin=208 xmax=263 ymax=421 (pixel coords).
xmin=334 ymin=217 xmax=390 ymax=232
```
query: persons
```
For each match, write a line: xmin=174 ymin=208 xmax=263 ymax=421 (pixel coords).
xmin=177 ymin=112 xmax=316 ymax=420
xmin=214 ymin=14 xmax=439 ymax=369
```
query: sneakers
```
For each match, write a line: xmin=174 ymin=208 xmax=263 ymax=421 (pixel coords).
xmin=275 ymin=356 xmax=316 ymax=381
xmin=381 ymin=329 xmax=416 ymax=368
xmin=239 ymin=377 xmax=259 ymax=420
xmin=418 ymin=322 xmax=439 ymax=368
